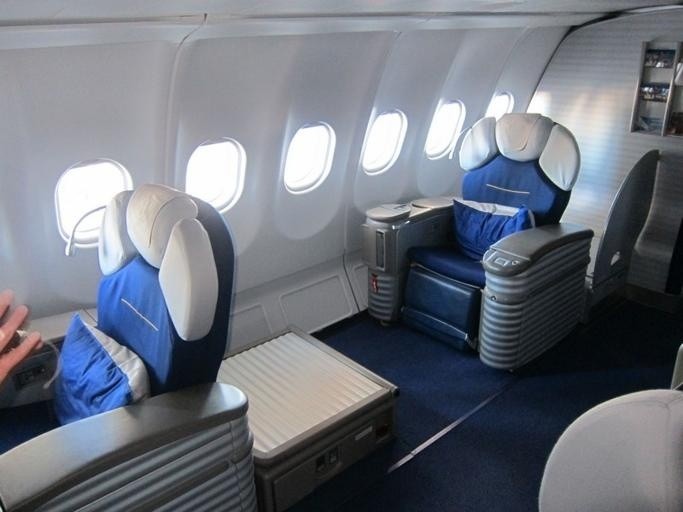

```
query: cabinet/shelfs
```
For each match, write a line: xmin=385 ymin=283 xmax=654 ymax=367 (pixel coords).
xmin=628 ymin=41 xmax=683 ymax=140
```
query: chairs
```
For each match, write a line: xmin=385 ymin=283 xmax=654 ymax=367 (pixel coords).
xmin=538 ymin=380 xmax=683 ymax=511
xmin=359 ymin=111 xmax=595 ymax=369
xmin=0 ymin=181 xmax=260 ymax=512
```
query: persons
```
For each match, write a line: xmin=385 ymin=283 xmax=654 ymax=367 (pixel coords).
xmin=0 ymin=288 xmax=41 ymax=382
